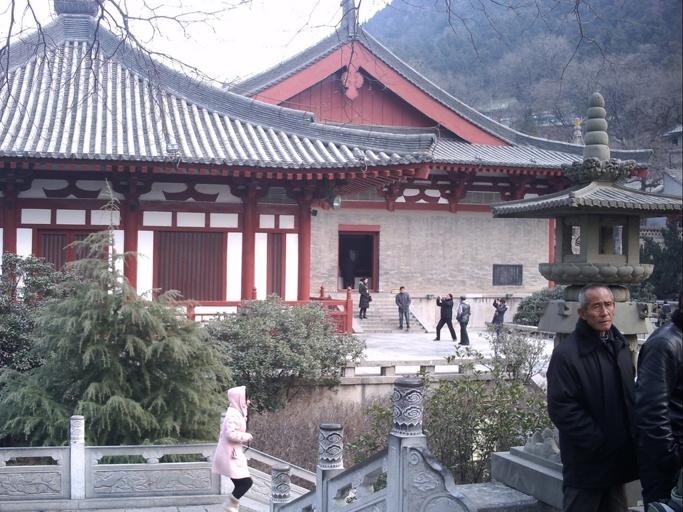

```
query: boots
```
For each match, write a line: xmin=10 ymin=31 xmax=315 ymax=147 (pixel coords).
xmin=221 ymin=495 xmax=240 ymax=512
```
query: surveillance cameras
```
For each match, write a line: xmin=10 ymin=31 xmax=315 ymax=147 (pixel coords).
xmin=311 ymin=209 xmax=318 ymax=217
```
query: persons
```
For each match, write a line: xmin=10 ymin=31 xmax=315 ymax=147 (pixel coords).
xmin=635 ymin=290 xmax=683 ymax=511
xmin=456 ymin=295 xmax=470 ymax=346
xmin=545 ymin=282 xmax=641 ymax=511
xmin=343 ymin=256 xmax=354 ymax=288
xmin=493 ymin=298 xmax=508 ymax=339
xmin=433 ymin=294 xmax=457 ymax=341
xmin=211 ymin=385 xmax=253 ymax=512
xmin=661 ymin=301 xmax=671 ymax=314
xmin=359 ymin=278 xmax=372 ymax=319
xmin=396 ymin=286 xmax=411 ymax=328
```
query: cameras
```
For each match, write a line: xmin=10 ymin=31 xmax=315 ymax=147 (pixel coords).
xmin=497 ymin=298 xmax=500 ymax=301
xmin=439 ymin=296 xmax=443 ymax=299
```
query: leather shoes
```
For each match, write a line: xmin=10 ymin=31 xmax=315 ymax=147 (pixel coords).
xmin=360 ymin=316 xmax=363 ymax=319
xmin=363 ymin=316 xmax=367 ymax=318
